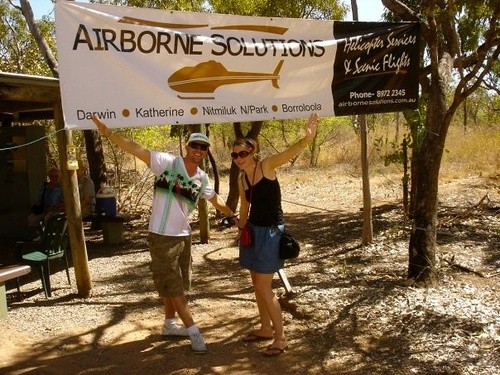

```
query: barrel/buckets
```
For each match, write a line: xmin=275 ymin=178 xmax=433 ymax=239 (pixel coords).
xmin=96 ymin=186 xmax=116 ymax=219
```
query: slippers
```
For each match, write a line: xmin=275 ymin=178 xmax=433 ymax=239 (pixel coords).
xmin=262 ymin=344 xmax=288 ymax=357
xmin=242 ymin=331 xmax=274 ymax=342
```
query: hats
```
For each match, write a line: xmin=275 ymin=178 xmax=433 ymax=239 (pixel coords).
xmin=188 ymin=133 xmax=210 ymax=147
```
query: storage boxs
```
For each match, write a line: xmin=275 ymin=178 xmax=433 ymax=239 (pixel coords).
xmin=95 ymin=187 xmax=118 ymax=216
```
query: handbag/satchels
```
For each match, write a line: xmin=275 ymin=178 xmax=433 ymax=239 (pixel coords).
xmin=279 ymin=232 xmax=300 ymax=260
xmin=239 ymin=223 xmax=252 ymax=249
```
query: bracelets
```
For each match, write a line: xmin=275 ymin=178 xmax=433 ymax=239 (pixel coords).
xmin=238 ymin=227 xmax=243 ymax=230
xmin=107 ymin=130 xmax=112 ymax=138
xmin=298 ymin=139 xmax=308 ymax=148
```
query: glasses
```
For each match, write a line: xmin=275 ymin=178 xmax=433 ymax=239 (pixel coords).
xmin=188 ymin=142 xmax=209 ymax=151
xmin=231 ymin=151 xmax=251 ymax=159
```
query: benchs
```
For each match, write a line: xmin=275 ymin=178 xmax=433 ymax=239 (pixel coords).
xmin=0 ymin=264 xmax=31 ymax=319
xmin=86 ymin=215 xmax=141 ymax=245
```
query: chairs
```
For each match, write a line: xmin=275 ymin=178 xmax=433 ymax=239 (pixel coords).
xmin=16 ymin=213 xmax=71 ymax=297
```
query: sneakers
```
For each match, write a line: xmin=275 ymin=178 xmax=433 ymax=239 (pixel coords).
xmin=161 ymin=324 xmax=189 ymax=337
xmin=189 ymin=331 xmax=208 ymax=355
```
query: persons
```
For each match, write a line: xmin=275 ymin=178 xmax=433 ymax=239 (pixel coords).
xmin=91 ymin=115 xmax=239 ymax=354
xmin=27 ymin=166 xmax=64 ymax=242
xmin=231 ymin=112 xmax=319 ymax=358
xmin=44 ymin=164 xmax=97 ymax=254
